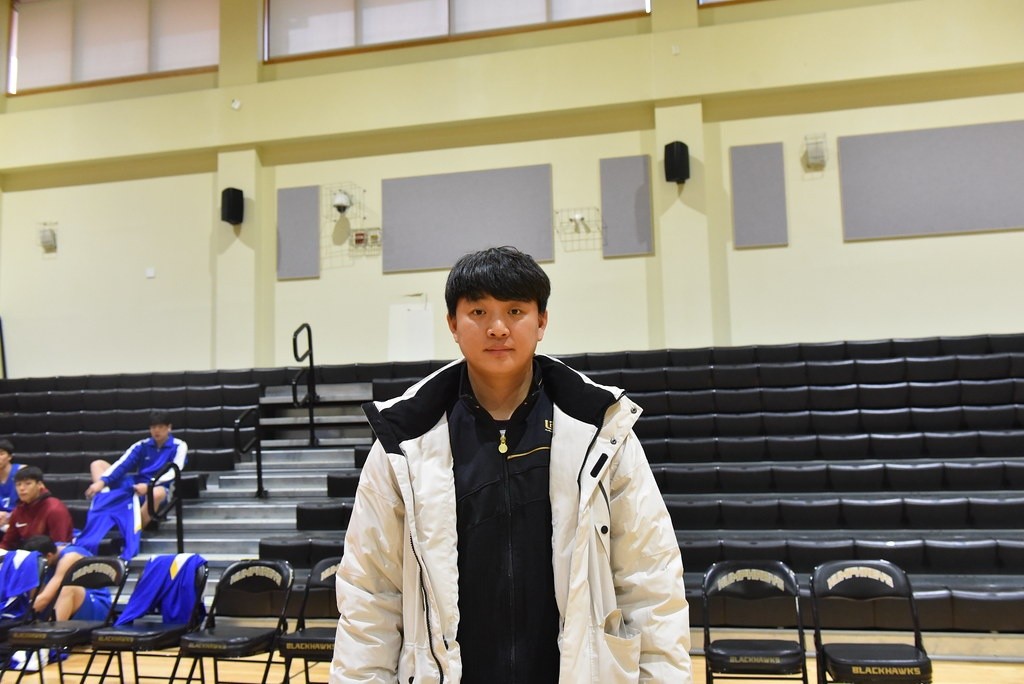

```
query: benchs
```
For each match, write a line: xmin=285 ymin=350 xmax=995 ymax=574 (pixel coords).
xmin=0 ymin=333 xmax=1023 ymax=633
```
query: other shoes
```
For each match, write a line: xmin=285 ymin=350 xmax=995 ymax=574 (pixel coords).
xmin=16 ymin=648 xmax=51 ymax=671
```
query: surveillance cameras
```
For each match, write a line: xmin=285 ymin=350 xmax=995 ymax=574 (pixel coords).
xmin=333 ymin=193 xmax=350 ymax=213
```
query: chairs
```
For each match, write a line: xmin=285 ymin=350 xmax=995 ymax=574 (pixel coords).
xmin=0 ymin=553 xmax=44 ymax=684
xmin=701 ymin=558 xmax=809 ymax=684
xmin=3 ymin=556 xmax=123 ymax=684
xmin=281 ymin=557 xmax=343 ymax=684
xmin=80 ymin=552 xmax=210 ymax=684
xmin=809 ymin=560 xmax=932 ymax=684
xmin=168 ymin=558 xmax=295 ymax=684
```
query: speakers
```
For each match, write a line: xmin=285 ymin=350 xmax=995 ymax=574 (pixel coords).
xmin=221 ymin=188 xmax=244 ymax=225
xmin=664 ymin=142 xmax=689 ymax=184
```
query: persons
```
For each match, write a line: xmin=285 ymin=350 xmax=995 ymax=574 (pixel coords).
xmin=330 ymin=245 xmax=693 ymax=684
xmin=0 ymin=410 xmax=191 ymax=673
